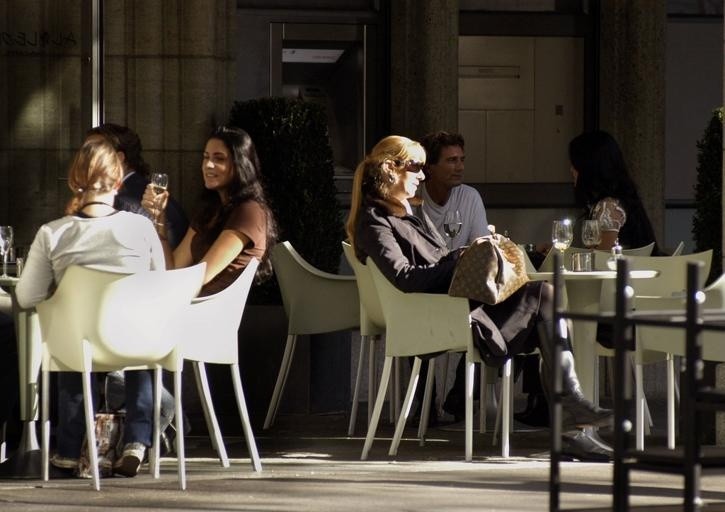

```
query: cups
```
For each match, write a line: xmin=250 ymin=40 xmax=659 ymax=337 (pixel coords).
xmin=570 ymin=251 xmax=595 ymax=272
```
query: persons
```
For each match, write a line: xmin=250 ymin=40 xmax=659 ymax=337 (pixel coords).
xmin=101 ymin=127 xmax=277 ymax=463
xmin=346 ymin=135 xmax=617 ymax=462
xmin=88 ymin=124 xmax=188 ymax=251
xmin=408 ymin=131 xmax=493 ymax=426
xmin=512 ymin=131 xmax=659 ymax=426
xmin=14 ymin=137 xmax=166 ymax=477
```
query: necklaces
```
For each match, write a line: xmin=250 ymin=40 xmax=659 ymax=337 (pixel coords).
xmin=77 ymin=201 xmax=116 ymax=213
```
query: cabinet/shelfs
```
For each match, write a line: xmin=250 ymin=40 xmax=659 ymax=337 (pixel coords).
xmin=458 ymin=36 xmax=584 ymax=183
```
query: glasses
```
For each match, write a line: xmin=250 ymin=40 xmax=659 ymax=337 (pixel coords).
xmin=405 ymin=162 xmax=423 ymax=174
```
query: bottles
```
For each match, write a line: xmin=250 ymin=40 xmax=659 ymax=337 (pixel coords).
xmin=607 ymin=246 xmax=627 ymax=271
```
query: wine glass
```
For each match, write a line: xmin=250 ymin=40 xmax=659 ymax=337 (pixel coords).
xmin=551 ymin=219 xmax=574 ymax=272
xmin=443 ymin=209 xmax=463 ymax=251
xmin=151 ymin=172 xmax=168 ymax=225
xmin=580 ymin=218 xmax=603 ymax=248
xmin=0 ymin=226 xmax=14 ymax=279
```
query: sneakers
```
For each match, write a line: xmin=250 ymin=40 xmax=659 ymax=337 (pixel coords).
xmin=49 ymin=452 xmax=79 ymax=469
xmin=413 ymin=414 xmax=456 ymax=426
xmin=114 ymin=442 xmax=146 ymax=476
xmin=160 ymin=434 xmax=172 ymax=457
xmin=165 ymin=413 xmax=191 ymax=437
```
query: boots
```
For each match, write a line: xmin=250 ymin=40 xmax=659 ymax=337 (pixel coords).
xmin=536 ymin=316 xmax=614 ymax=426
xmin=561 ymin=426 xmax=614 ymax=462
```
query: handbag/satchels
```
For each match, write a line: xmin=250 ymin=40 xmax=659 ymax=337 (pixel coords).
xmin=448 ymin=235 xmax=535 ymax=306
xmin=76 ymin=414 xmax=126 ymax=478
